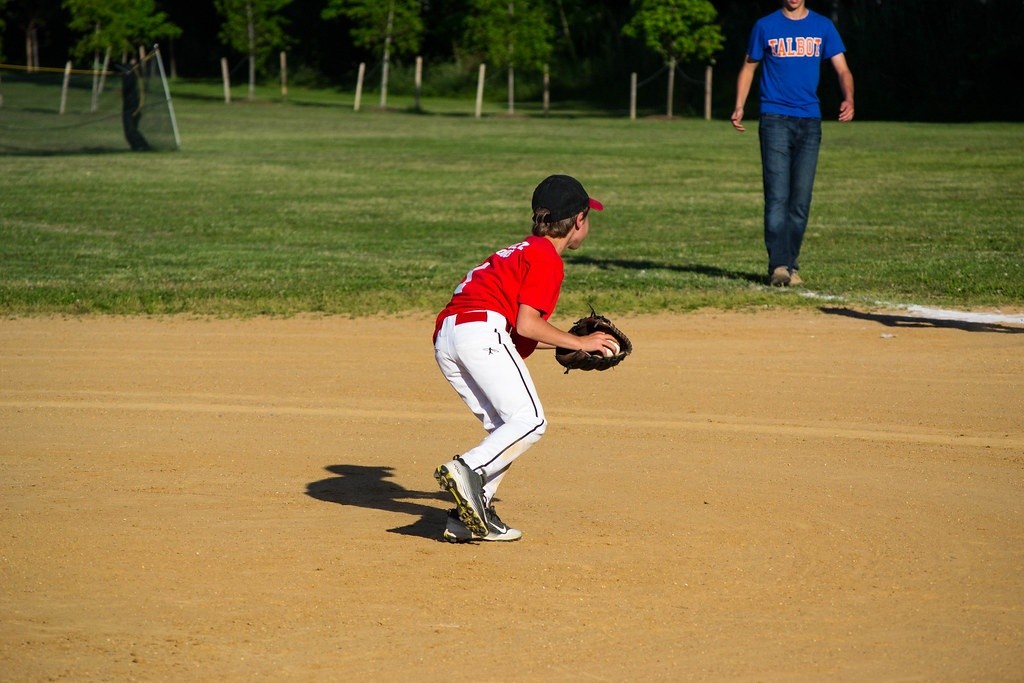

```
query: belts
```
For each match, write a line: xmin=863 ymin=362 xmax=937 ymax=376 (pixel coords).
xmin=456 ymin=312 xmax=509 ymax=332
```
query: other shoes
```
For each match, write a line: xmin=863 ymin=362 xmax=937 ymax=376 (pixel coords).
xmin=771 ymin=267 xmax=791 ymax=286
xmin=789 ymin=268 xmax=803 ymax=286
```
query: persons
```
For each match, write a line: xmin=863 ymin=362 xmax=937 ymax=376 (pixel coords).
xmin=730 ymin=0 xmax=855 ymax=286
xmin=121 ymin=58 xmax=153 ymax=153
xmin=432 ymin=174 xmax=621 ymax=544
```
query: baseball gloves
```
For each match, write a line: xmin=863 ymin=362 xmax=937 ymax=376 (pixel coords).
xmin=555 ymin=314 xmax=633 ymax=372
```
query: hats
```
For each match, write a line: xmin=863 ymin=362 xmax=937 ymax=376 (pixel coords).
xmin=532 ymin=175 xmax=605 ymax=223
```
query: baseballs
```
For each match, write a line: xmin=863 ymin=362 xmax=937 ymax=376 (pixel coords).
xmin=601 ymin=338 xmax=620 ymax=357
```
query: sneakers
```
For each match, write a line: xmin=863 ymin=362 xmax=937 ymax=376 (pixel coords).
xmin=442 ymin=507 xmax=521 ymax=543
xmin=434 ymin=454 xmax=489 ymax=537
